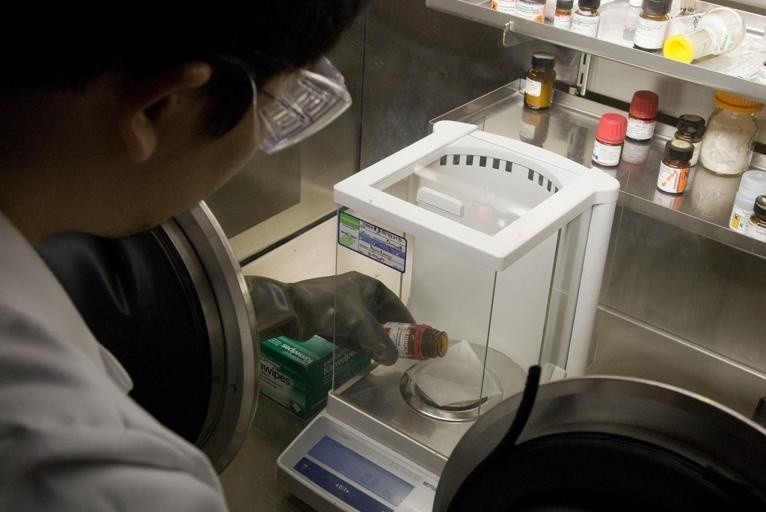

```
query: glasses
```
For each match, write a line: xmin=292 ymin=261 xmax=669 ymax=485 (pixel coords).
xmin=224 ymin=52 xmax=352 ymax=155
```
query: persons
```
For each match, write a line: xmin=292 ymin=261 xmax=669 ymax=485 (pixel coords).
xmin=0 ymin=0 xmax=415 ymax=511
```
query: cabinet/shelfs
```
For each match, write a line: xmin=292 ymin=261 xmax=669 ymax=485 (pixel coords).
xmin=424 ymin=0 xmax=766 ymax=260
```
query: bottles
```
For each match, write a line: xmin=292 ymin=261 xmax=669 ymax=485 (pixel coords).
xmin=492 ymin=0 xmax=671 ymax=53
xmin=523 ymin=56 xmax=555 ymax=109
xmin=664 ymin=8 xmax=745 ymax=66
xmin=384 ymin=322 xmax=448 ymax=360
xmin=592 ymin=90 xmax=765 ymax=242
xmin=519 ymin=110 xmax=549 ymax=149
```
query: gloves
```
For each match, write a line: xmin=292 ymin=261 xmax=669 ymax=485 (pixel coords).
xmin=243 ymin=270 xmax=417 ymax=366
